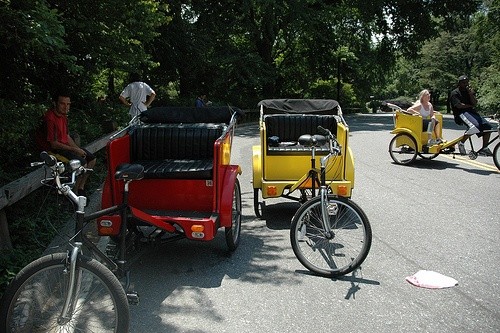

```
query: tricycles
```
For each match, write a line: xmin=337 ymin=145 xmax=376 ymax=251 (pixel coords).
xmin=0 ymin=104 xmax=242 ymax=333
xmin=382 ymin=100 xmax=500 ymax=171
xmin=250 ymin=99 xmax=372 ymax=278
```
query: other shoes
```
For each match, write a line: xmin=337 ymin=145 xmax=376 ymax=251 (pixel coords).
xmin=457 ymin=140 xmax=466 ymax=155
xmin=479 ymin=147 xmax=494 ymax=156
xmin=428 ymin=138 xmax=448 ymax=144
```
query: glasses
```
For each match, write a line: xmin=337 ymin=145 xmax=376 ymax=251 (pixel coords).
xmin=57 ymin=102 xmax=72 ymax=108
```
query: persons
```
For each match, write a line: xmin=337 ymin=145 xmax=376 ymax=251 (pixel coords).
xmin=450 ymin=76 xmax=492 ymax=155
xmin=407 ymin=90 xmax=447 ymax=145
xmin=41 ymin=94 xmax=96 ymax=206
xmin=196 ymin=93 xmax=208 ymax=108
xmin=119 ymin=73 xmax=156 ymax=123
